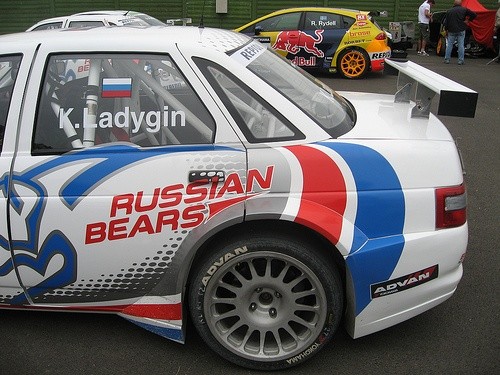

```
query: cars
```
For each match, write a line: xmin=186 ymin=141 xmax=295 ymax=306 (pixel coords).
xmin=0 ymin=8 xmax=234 ymax=92
xmin=0 ymin=24 xmax=479 ymax=371
xmin=230 ymin=7 xmax=392 ymax=80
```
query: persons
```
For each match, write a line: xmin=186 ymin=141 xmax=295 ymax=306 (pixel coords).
xmin=413 ymin=0 xmax=477 ymax=65
xmin=492 ymin=0 xmax=500 ymax=62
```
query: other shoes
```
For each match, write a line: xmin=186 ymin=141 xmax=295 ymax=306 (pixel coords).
xmin=421 ymin=51 xmax=429 ymax=56
xmin=417 ymin=51 xmax=421 ymax=54
xmin=444 ymin=59 xmax=450 ymax=64
xmin=457 ymin=60 xmax=464 ymax=65
